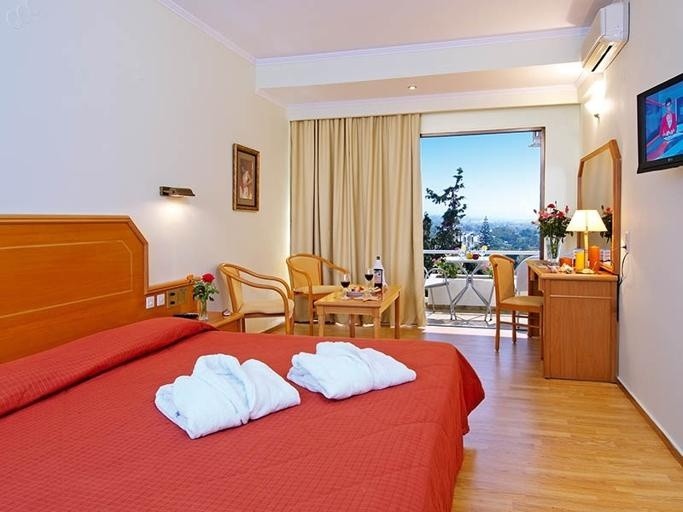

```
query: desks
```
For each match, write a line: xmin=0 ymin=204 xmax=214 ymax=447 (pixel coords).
xmin=443 ymin=257 xmax=492 ymax=321
xmin=313 ymin=286 xmax=400 ymax=339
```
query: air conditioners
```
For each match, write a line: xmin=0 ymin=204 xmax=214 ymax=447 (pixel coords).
xmin=580 ymin=0 xmax=629 ymax=74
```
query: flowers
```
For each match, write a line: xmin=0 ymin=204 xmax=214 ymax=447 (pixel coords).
xmin=531 ymin=201 xmax=573 ymax=258
xmin=432 ymin=243 xmax=494 ymax=278
xmin=598 ymin=204 xmax=613 ymax=243
xmin=187 ymin=273 xmax=219 ymax=313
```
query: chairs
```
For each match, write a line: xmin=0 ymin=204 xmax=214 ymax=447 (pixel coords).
xmin=485 ymin=256 xmax=539 ymax=321
xmin=286 ymin=253 xmax=349 ymax=337
xmin=423 ymin=267 xmax=457 ymax=320
xmin=218 ymin=263 xmax=295 ymax=335
xmin=489 ymin=254 xmax=544 ymax=360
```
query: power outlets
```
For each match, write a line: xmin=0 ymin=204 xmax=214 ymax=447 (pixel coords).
xmin=625 ymin=231 xmax=630 ymax=253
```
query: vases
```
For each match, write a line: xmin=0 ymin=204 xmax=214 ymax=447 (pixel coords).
xmin=546 ymin=237 xmax=561 ymax=267
xmin=426 ymin=274 xmax=518 ymax=307
xmin=196 ymin=300 xmax=208 ymax=321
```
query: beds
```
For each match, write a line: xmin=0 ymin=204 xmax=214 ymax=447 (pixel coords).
xmin=0 ymin=215 xmax=485 ymax=512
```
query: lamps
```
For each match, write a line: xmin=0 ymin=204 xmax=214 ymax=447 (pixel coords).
xmin=566 ymin=210 xmax=608 ymax=274
xmin=160 ymin=186 xmax=195 ymax=197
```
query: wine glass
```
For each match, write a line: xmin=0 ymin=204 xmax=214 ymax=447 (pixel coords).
xmin=458 ymin=243 xmax=487 ymax=260
xmin=342 ymin=255 xmax=386 ymax=300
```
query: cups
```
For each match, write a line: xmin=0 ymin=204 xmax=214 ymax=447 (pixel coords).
xmin=574 ymin=249 xmax=585 ymax=271
xmin=589 ymin=245 xmax=601 ymax=272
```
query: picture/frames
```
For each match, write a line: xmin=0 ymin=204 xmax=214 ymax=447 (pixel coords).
xmin=232 ymin=143 xmax=259 ymax=211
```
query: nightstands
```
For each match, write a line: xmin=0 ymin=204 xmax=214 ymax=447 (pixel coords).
xmin=191 ymin=310 xmax=244 ymax=332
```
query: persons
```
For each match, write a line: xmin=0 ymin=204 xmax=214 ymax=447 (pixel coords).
xmin=661 ymin=97 xmax=677 ymax=137
xmin=241 ymin=164 xmax=252 ymax=199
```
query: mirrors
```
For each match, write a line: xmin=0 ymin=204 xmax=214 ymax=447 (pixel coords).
xmin=577 ymin=139 xmax=621 ymax=274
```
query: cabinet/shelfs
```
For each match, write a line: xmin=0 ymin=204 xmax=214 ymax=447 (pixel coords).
xmin=526 ymin=260 xmax=618 ymax=383
